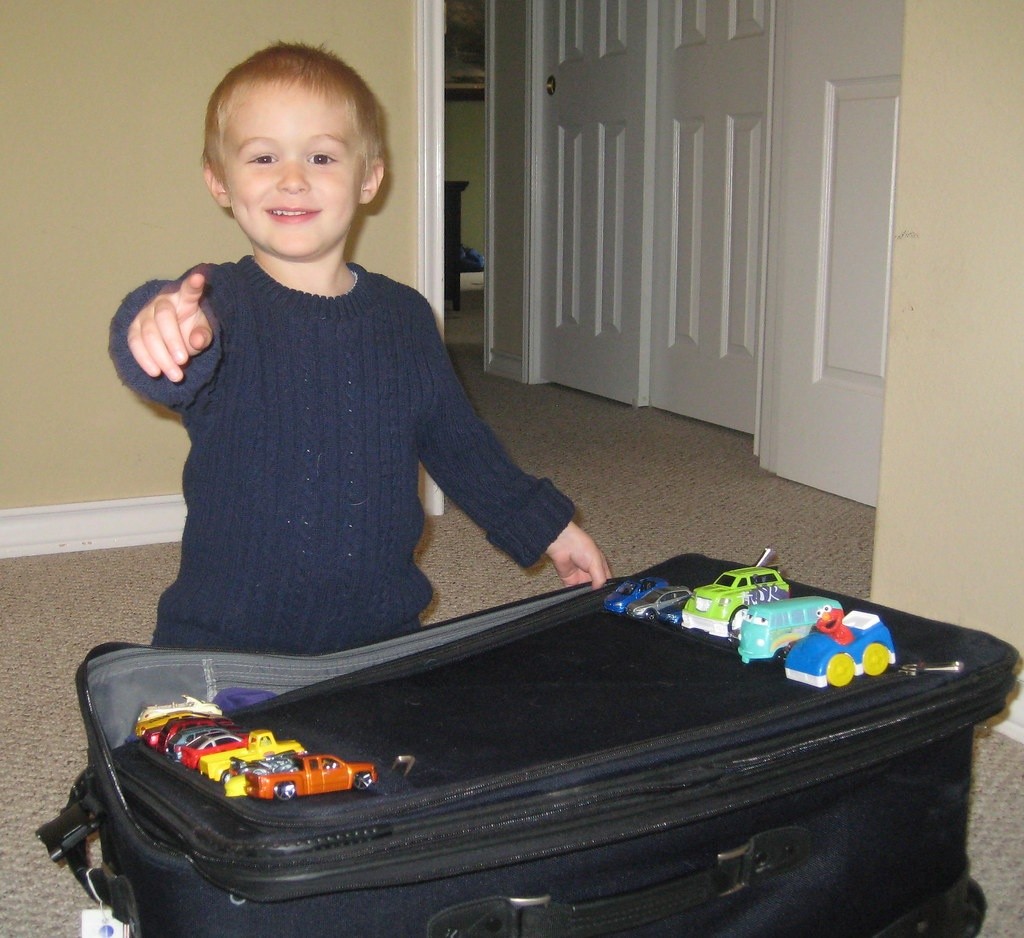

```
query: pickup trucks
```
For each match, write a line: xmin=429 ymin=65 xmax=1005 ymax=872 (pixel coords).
xmin=199 ymin=729 xmax=303 ymax=798
xmin=249 ymin=756 xmax=379 ymax=802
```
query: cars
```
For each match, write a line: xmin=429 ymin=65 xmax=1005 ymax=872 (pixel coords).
xmin=603 ymin=575 xmax=692 ymax=628
xmin=782 ymin=609 xmax=897 ymax=689
xmin=135 ymin=694 xmax=260 ymax=771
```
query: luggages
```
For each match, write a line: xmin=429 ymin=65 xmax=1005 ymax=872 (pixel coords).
xmin=37 ymin=552 xmax=1019 ymax=938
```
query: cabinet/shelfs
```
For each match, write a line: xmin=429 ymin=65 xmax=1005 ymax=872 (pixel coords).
xmin=445 ymin=180 xmax=469 ymax=311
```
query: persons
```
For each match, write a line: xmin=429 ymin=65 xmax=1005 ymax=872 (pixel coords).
xmin=110 ymin=46 xmax=612 ymax=655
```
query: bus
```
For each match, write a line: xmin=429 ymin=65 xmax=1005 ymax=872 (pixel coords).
xmin=736 ymin=595 xmax=844 ymax=672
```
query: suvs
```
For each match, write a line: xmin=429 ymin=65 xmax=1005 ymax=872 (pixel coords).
xmin=679 ymin=565 xmax=791 ymax=649
